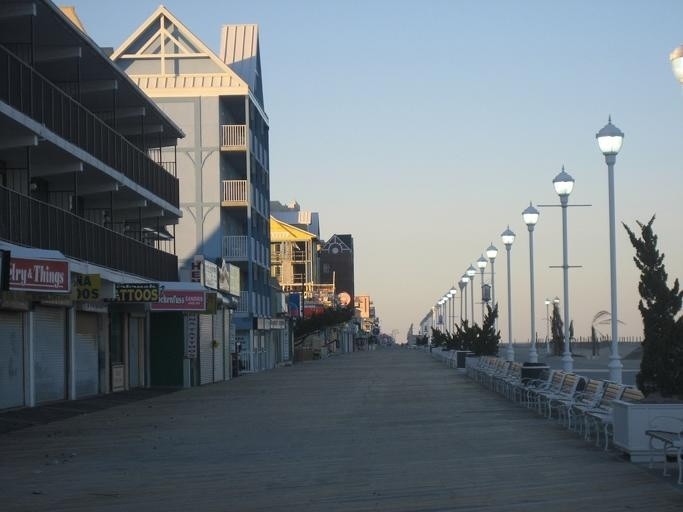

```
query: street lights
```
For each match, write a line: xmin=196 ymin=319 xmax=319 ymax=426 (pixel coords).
xmin=419 ymin=224 xmax=497 ymax=361
xmin=595 ymin=114 xmax=626 ymax=387
xmin=501 ymin=223 xmax=516 ymax=362
xmin=539 ymin=165 xmax=588 ymax=375
xmin=521 ymin=198 xmax=542 ymax=370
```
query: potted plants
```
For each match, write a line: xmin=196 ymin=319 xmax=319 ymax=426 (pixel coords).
xmin=611 ymin=213 xmax=682 ymax=462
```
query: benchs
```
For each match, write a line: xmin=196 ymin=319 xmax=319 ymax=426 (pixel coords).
xmin=644 ymin=428 xmax=682 ymax=483
xmin=466 ymin=355 xmax=645 ymax=454
